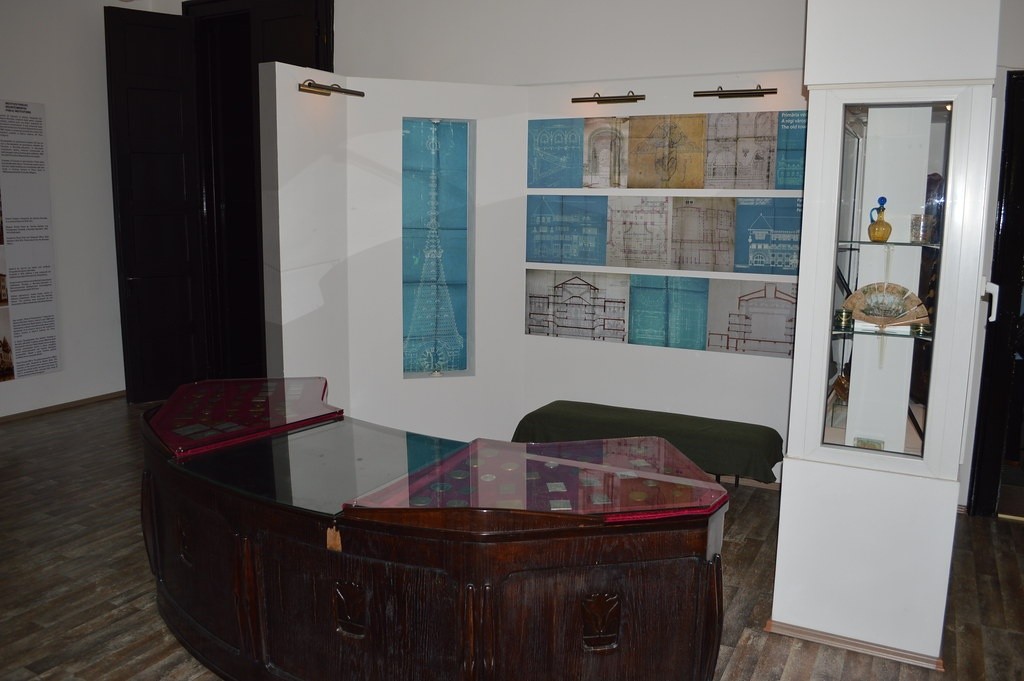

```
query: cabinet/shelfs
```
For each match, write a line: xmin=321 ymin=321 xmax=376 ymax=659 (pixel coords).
xmin=837 ymin=238 xmax=942 ymax=340
xmin=140 ymin=376 xmax=733 ymax=681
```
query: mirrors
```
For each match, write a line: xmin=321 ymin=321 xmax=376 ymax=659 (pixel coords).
xmin=820 ymin=100 xmax=954 ymax=460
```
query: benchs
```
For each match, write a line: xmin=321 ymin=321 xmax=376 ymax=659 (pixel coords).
xmin=512 ymin=398 xmax=784 ymax=488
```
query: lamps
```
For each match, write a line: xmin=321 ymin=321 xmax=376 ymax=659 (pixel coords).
xmin=298 ymin=78 xmax=366 ymax=98
xmin=570 ymin=91 xmax=647 ymax=104
xmin=693 ymin=84 xmax=778 ymax=99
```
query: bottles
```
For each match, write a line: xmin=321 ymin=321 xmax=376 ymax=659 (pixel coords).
xmin=868 ymin=196 xmax=892 ymax=243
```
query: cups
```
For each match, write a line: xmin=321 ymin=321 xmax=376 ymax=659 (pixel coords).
xmin=910 ymin=213 xmax=936 ymax=245
